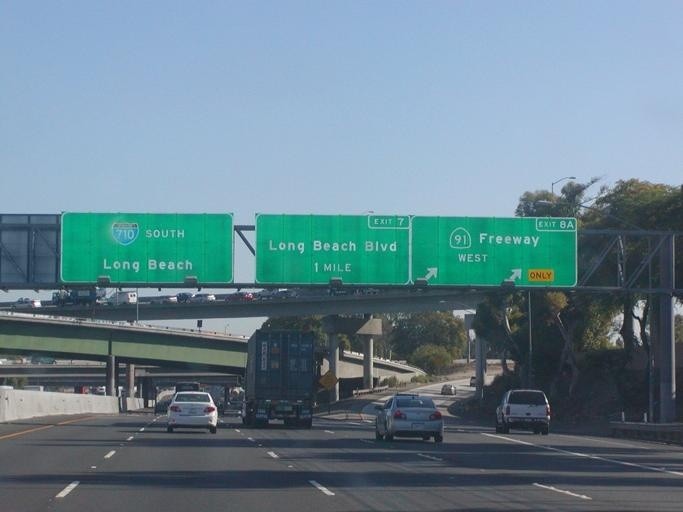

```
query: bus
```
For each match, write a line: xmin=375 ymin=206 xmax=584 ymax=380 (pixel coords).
xmin=176 ymin=382 xmax=201 ymax=393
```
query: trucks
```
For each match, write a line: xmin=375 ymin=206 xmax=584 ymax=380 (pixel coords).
xmin=224 ymin=386 xmax=245 ymax=416
xmin=236 ymin=330 xmax=315 ymax=427
xmin=52 ymin=288 xmax=106 ymax=308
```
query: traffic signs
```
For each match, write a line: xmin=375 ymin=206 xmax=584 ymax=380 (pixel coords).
xmin=411 ymin=215 xmax=577 ymax=288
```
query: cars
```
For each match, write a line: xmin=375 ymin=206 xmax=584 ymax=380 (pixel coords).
xmin=470 ymin=376 xmax=477 ymax=387
xmin=375 ymin=392 xmax=443 ymax=442
xmin=440 ymin=384 xmax=457 ymax=395
xmin=11 ymin=297 xmax=42 ymax=310
xmin=495 ymin=389 xmax=551 ymax=435
xmin=225 ymin=290 xmax=297 ymax=302
xmin=156 ymin=391 xmax=222 ymax=433
xmin=149 ymin=293 xmax=216 ymax=304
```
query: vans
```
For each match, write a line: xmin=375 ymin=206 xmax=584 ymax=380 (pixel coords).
xmin=107 ymin=291 xmax=137 ymax=305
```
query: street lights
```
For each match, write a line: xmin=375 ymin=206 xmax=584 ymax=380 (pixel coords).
xmin=551 ymin=176 xmax=576 ymax=195
xmin=534 ymin=199 xmax=656 ymax=422
